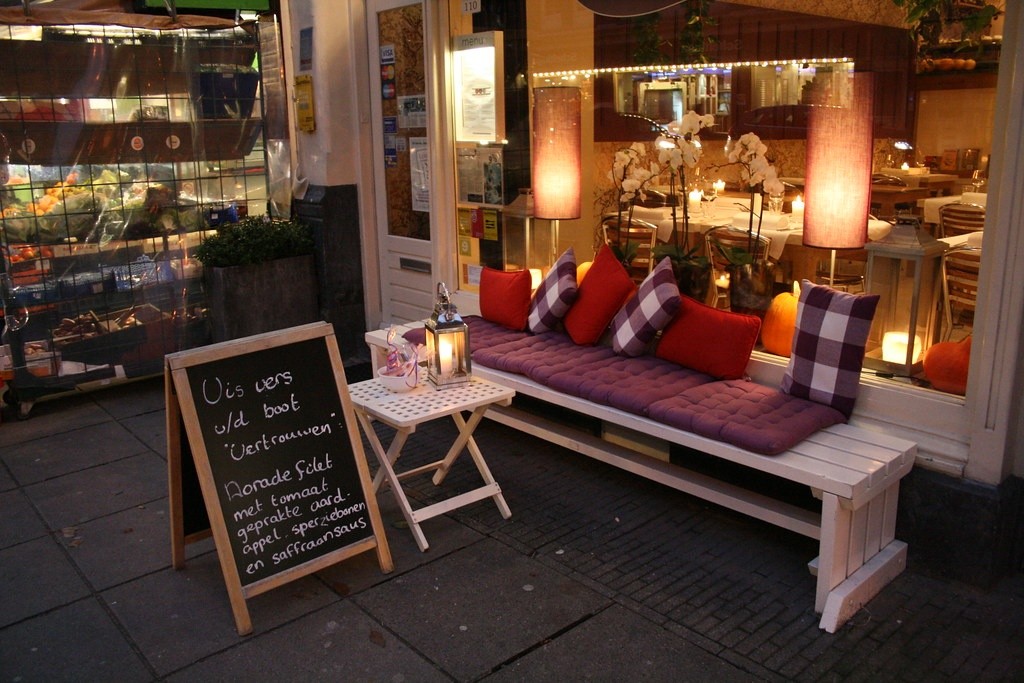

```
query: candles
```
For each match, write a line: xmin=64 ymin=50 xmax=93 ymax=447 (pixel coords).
xmin=689 ymin=189 xmax=701 ymax=211
xmin=717 ymin=180 xmax=725 ymax=194
xmin=882 ymin=331 xmax=922 ymax=364
xmin=792 ymin=195 xmax=804 ymax=216
xmin=901 ymin=162 xmax=909 ymax=171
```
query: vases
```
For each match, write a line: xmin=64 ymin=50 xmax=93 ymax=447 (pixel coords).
xmin=730 ymin=258 xmax=773 ymax=316
xmin=655 ymin=257 xmax=712 ymax=303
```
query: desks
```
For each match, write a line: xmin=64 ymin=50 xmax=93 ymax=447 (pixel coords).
xmin=600 ymin=167 xmax=988 ymax=344
xmin=347 ymin=367 xmax=515 ymax=551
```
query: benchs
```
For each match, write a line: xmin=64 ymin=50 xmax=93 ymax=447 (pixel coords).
xmin=365 ymin=314 xmax=918 ymax=632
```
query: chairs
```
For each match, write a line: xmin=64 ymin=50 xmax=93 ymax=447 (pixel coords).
xmin=939 ymin=203 xmax=986 ymax=238
xmin=602 ymin=215 xmax=657 ymax=284
xmin=705 ymin=225 xmax=771 ymax=308
xmin=941 ymin=249 xmax=981 ymax=343
xmin=817 ymin=248 xmax=868 ymax=297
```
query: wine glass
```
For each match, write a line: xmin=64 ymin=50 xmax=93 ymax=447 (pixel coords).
xmin=703 ymin=181 xmax=717 ymax=202
xmin=769 ymin=192 xmax=784 ymax=212
xmin=972 ymin=170 xmax=985 ymax=193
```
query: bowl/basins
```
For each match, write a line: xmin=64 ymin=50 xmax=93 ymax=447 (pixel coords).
xmin=378 ymin=365 xmax=423 ymax=389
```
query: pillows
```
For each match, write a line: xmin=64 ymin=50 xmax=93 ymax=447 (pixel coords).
xmin=478 ymin=244 xmax=880 ymax=420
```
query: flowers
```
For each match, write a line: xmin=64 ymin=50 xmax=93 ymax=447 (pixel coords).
xmin=608 ymin=110 xmax=785 ymax=298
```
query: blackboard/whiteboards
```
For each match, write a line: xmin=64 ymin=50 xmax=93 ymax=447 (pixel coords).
xmin=164 ymin=321 xmax=387 ymax=600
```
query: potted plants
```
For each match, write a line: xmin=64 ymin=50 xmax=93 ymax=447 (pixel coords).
xmin=191 ymin=214 xmax=316 ymax=342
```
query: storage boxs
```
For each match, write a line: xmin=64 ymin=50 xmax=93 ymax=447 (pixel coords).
xmin=0 ymin=227 xmax=216 ymax=396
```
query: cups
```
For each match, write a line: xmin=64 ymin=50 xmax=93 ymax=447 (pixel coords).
xmin=962 ymin=185 xmax=977 ymax=192
xmin=702 ymin=201 xmax=715 ymax=221
xmin=921 ymin=167 xmax=930 ymax=175
xmin=769 ymin=198 xmax=783 ymax=214
